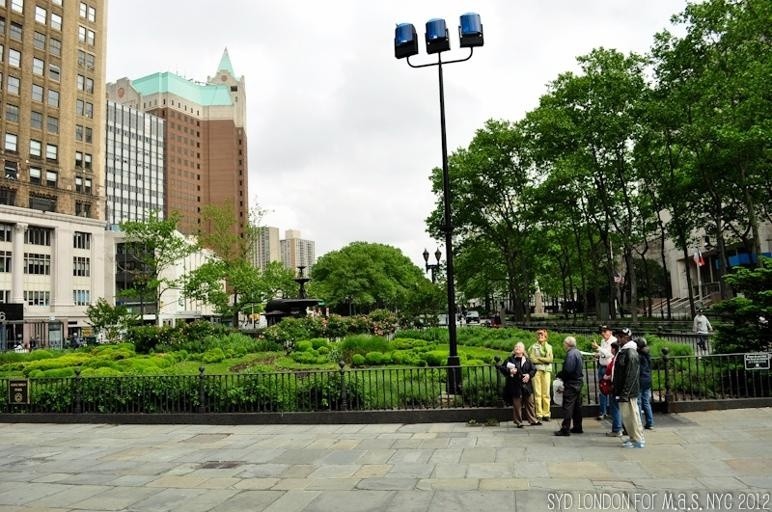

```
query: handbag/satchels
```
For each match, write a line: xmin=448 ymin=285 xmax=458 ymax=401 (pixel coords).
xmin=599 ymin=379 xmax=610 ymax=396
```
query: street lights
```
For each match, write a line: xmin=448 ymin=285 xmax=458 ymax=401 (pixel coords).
xmin=390 ymin=9 xmax=488 ymax=396
xmin=420 ymin=246 xmax=443 ymax=288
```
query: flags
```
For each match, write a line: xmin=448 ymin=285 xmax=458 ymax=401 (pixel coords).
xmin=693 ymin=248 xmax=704 ymax=267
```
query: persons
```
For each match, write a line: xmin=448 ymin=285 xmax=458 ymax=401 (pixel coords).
xmin=498 ymin=341 xmax=544 ymax=428
xmin=17 ymin=332 xmax=41 ymax=354
xmin=691 ymin=308 xmax=714 ymax=352
xmin=591 ymin=324 xmax=618 ymax=421
xmin=73 ymin=333 xmax=82 ymax=350
xmin=322 ymin=316 xmax=329 ymax=327
xmin=525 ymin=327 xmax=553 ymax=422
xmin=612 ymin=326 xmax=646 ymax=449
xmin=550 ymin=336 xmax=585 ymax=436
xmin=632 ymin=336 xmax=655 ymax=431
xmin=601 ymin=340 xmax=627 ymax=437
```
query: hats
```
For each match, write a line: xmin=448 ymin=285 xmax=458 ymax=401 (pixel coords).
xmin=613 ymin=327 xmax=632 ymax=336
xmin=601 ymin=325 xmax=611 ymax=330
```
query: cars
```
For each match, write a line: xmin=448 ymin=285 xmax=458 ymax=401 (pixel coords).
xmin=465 ymin=310 xmax=481 ymax=325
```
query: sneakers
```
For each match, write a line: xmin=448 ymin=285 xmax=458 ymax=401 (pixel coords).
xmin=554 ymin=428 xmax=582 ymax=436
xmin=517 ymin=415 xmax=550 ymax=427
xmin=598 ymin=414 xmax=608 ymax=420
xmin=606 ymin=425 xmax=653 ymax=447
